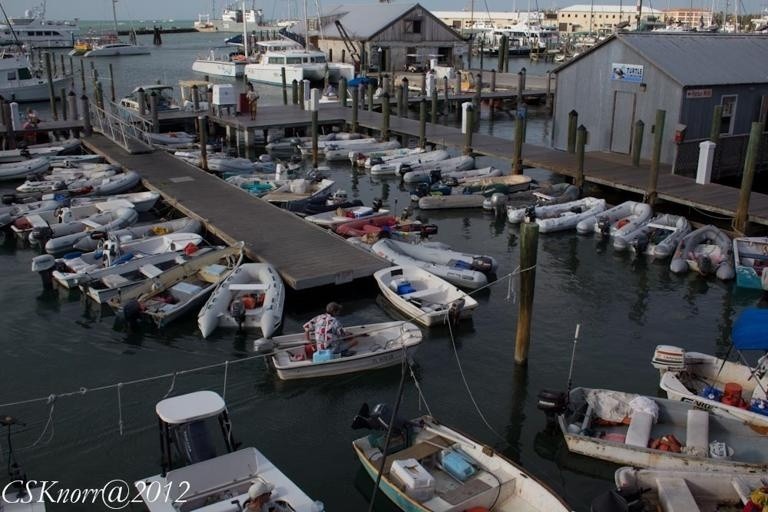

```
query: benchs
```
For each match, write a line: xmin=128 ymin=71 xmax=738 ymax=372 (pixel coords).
xmin=26 ymin=213 xmax=48 ymax=230
xmin=61 ymin=252 xmax=96 ymax=274
xmin=229 ymin=283 xmax=269 ymax=292
xmin=80 ymin=218 xmax=100 ymax=228
xmin=196 ymin=261 xmax=229 ymax=280
xmin=174 ymin=279 xmax=199 ymax=297
xmin=655 ymin=477 xmax=701 ymax=512
xmin=402 ymin=287 xmax=443 ymax=303
xmin=188 ymin=489 xmax=274 ymax=512
xmin=624 ymin=398 xmax=710 ymax=457
xmin=141 ymin=262 xmax=160 ymax=279
xmin=103 ymin=272 xmax=128 ymax=289
xmin=372 ymin=434 xmax=513 ymax=509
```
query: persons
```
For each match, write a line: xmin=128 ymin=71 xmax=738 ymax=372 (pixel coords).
xmin=302 ymin=302 xmax=358 ymax=357
xmin=23 ymin=108 xmax=40 ymax=128
xmin=324 ymin=80 xmax=335 ymax=95
xmin=275 ymin=159 xmax=288 ymax=180
xmin=246 ymin=86 xmax=259 ymax=120
xmin=51 ymin=180 xmax=68 ymax=199
xmin=242 ymin=482 xmax=288 ymax=512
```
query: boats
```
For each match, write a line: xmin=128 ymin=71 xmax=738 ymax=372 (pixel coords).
xmin=132 ymin=388 xmax=332 ymax=512
xmin=575 ymin=199 xmax=654 ymax=239
xmin=473 ymin=0 xmax=615 ymax=63
xmin=589 ymin=466 xmax=767 ymax=512
xmin=669 ymin=223 xmax=736 ymax=280
xmin=350 ymin=400 xmax=575 ymax=512
xmin=189 ymin=0 xmax=357 ymax=91
xmin=195 ymin=261 xmax=286 ymax=340
xmin=612 ymin=213 xmax=692 ymax=260
xmin=251 ymin=318 xmax=424 ymax=382
xmin=372 ymin=237 xmax=497 ymax=291
xmin=534 ymin=385 xmax=768 ymax=472
xmin=1 ymin=2 xmax=149 ymax=103
xmin=372 ymin=263 xmax=480 ymax=328
xmin=119 ymin=84 xmax=182 ymax=111
xmin=649 ymin=343 xmax=768 ymax=430
xmin=506 ymin=196 xmax=608 ymax=234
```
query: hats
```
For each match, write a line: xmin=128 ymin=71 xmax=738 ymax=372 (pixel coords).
xmin=249 ymin=481 xmax=275 ymax=501
xmin=326 ymin=301 xmax=344 ymax=314
